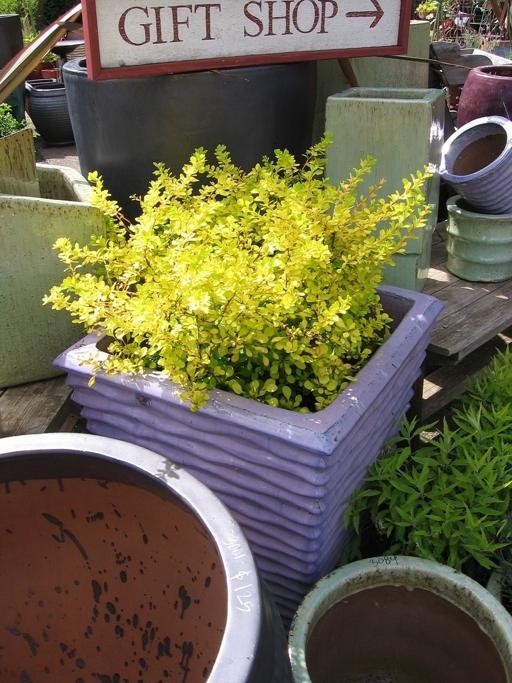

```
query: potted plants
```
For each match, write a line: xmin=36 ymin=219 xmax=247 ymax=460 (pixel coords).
xmin=41 ymin=129 xmax=444 ymax=627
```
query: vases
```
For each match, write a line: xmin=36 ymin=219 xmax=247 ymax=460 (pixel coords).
xmin=323 ymin=57 xmax=511 ymax=296
xmin=3 ymin=432 xmax=264 ymax=682
xmin=287 ymin=553 xmax=511 ymax=680
xmin=0 ymin=54 xmax=512 ymax=389
